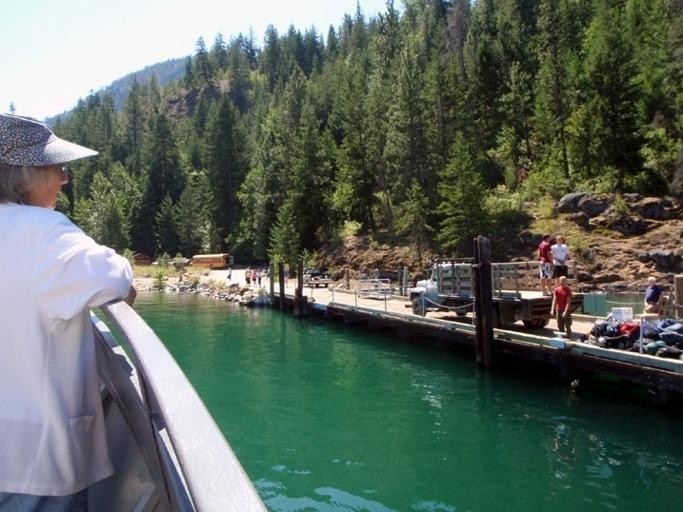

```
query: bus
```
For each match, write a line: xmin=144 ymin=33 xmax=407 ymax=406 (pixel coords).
xmin=190 ymin=253 xmax=230 ymax=270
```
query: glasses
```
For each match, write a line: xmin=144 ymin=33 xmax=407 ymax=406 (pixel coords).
xmin=54 ymin=163 xmax=69 ymax=177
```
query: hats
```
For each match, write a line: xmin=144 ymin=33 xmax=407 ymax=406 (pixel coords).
xmin=0 ymin=113 xmax=99 ymax=167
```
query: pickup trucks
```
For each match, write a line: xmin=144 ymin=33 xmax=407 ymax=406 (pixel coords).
xmin=300 ymin=267 xmax=333 ymax=288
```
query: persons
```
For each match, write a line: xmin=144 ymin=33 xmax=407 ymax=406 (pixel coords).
xmin=550 ymin=235 xmax=570 ymax=288
xmin=258 ymin=271 xmax=265 ymax=288
xmin=539 ymin=235 xmax=554 ymax=295
xmin=0 ymin=112 xmax=136 ymax=512
xmin=252 ymin=268 xmax=258 ymax=287
xmin=644 ymin=277 xmax=663 ymax=313
xmin=227 ymin=265 xmax=232 ymax=280
xmin=551 ymin=276 xmax=572 ymax=334
xmin=245 ymin=265 xmax=252 ymax=287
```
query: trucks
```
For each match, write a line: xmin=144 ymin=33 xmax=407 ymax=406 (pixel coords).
xmin=408 ymin=260 xmax=586 ymax=329
